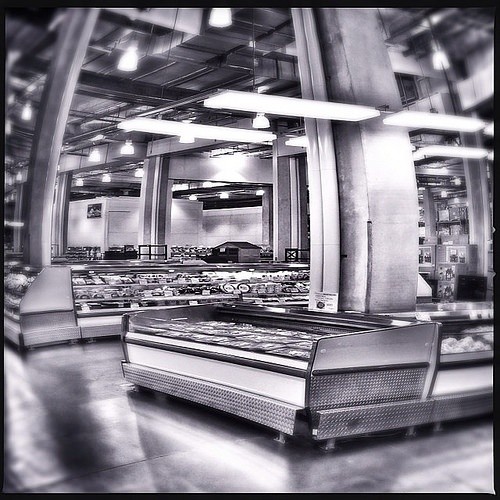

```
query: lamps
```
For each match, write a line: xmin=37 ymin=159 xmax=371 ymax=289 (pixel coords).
xmin=116 ymin=39 xmax=141 ymax=74
xmin=252 ymin=111 xmax=271 ymax=130
xmin=431 ymin=39 xmax=452 ymax=70
xmin=208 ymin=7 xmax=234 ymax=30
xmin=178 ymin=132 xmax=198 ymax=142
xmin=220 ymin=191 xmax=229 ymax=200
xmin=384 ymin=111 xmax=488 ymax=133
xmin=255 ymin=189 xmax=265 ymax=196
xmin=20 ymin=98 xmax=32 ymax=120
xmin=441 ymin=191 xmax=447 ymax=198
xmin=133 ymin=167 xmax=148 ymax=179
xmin=415 ymin=145 xmax=486 ymax=162
xmin=202 ymin=90 xmax=381 ymax=124
xmin=117 ymin=117 xmax=277 ymax=145
xmin=187 ymin=195 xmax=198 ymax=202
xmin=284 ymin=134 xmax=305 ymax=149
xmin=118 ymin=139 xmax=135 ymax=156
xmin=99 ymin=173 xmax=112 ymax=183
xmin=455 ymin=178 xmax=461 ymax=186
xmin=4 ymin=117 xmax=13 ymax=135
xmin=86 ymin=148 xmax=101 ymax=163
xmin=15 ymin=170 xmax=23 ymax=184
xmin=76 ymin=178 xmax=84 ymax=188
